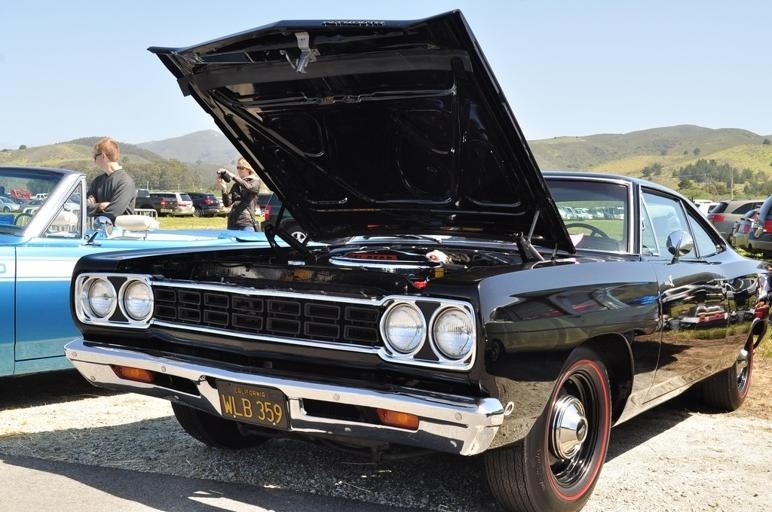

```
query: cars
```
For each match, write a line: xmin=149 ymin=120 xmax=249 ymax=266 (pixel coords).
xmin=483 ymin=286 xmax=660 ymax=362
xmin=554 ymin=202 xmax=623 ymax=223
xmin=690 ymin=194 xmax=772 ymax=256
xmin=523 ymin=274 xmax=771 ymax=335
xmin=60 ymin=10 xmax=770 ymax=511
xmin=1 ymin=166 xmax=330 ymax=376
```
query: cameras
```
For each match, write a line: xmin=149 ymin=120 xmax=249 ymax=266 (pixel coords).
xmin=217 ymin=169 xmax=222 ymax=177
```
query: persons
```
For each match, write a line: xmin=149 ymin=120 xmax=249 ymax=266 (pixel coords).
xmin=86 ymin=138 xmax=137 ymax=226
xmin=217 ymin=156 xmax=260 ymax=232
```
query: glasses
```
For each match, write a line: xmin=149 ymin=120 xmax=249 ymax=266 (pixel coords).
xmin=93 ymin=152 xmax=104 ymax=161
